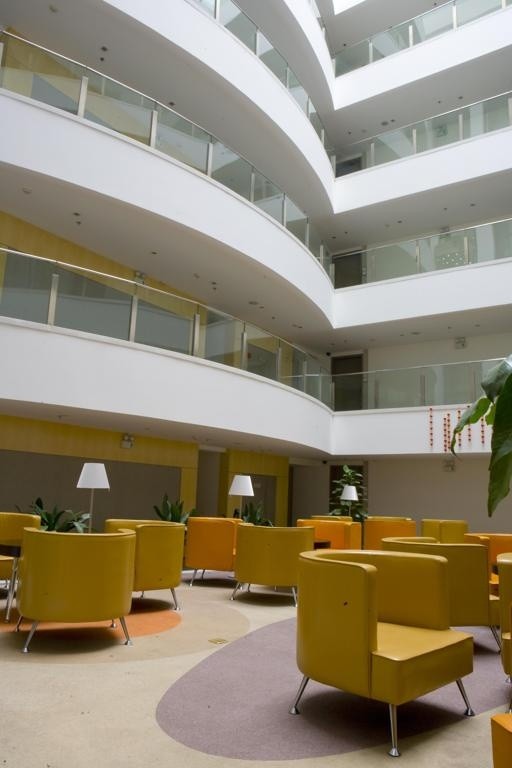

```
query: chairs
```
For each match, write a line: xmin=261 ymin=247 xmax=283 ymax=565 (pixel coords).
xmin=289 ymin=514 xmax=512 ymax=757
xmin=0 ymin=512 xmax=185 ymax=653
xmin=185 ymin=516 xmax=316 ymax=607
xmin=490 ymin=712 xmax=512 ymax=768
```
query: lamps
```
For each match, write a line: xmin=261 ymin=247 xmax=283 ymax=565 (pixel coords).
xmin=131 ymin=269 xmax=147 ymax=284
xmin=120 ymin=433 xmax=135 ymax=449
xmin=74 ymin=459 xmax=110 ymax=535
xmin=227 ymin=472 xmax=257 ymax=519
xmin=436 ymin=123 xmax=447 ymax=136
xmin=455 ymin=337 xmax=466 ymax=349
xmin=340 ymin=482 xmax=361 ymax=515
xmin=440 ymin=226 xmax=451 ymax=237
